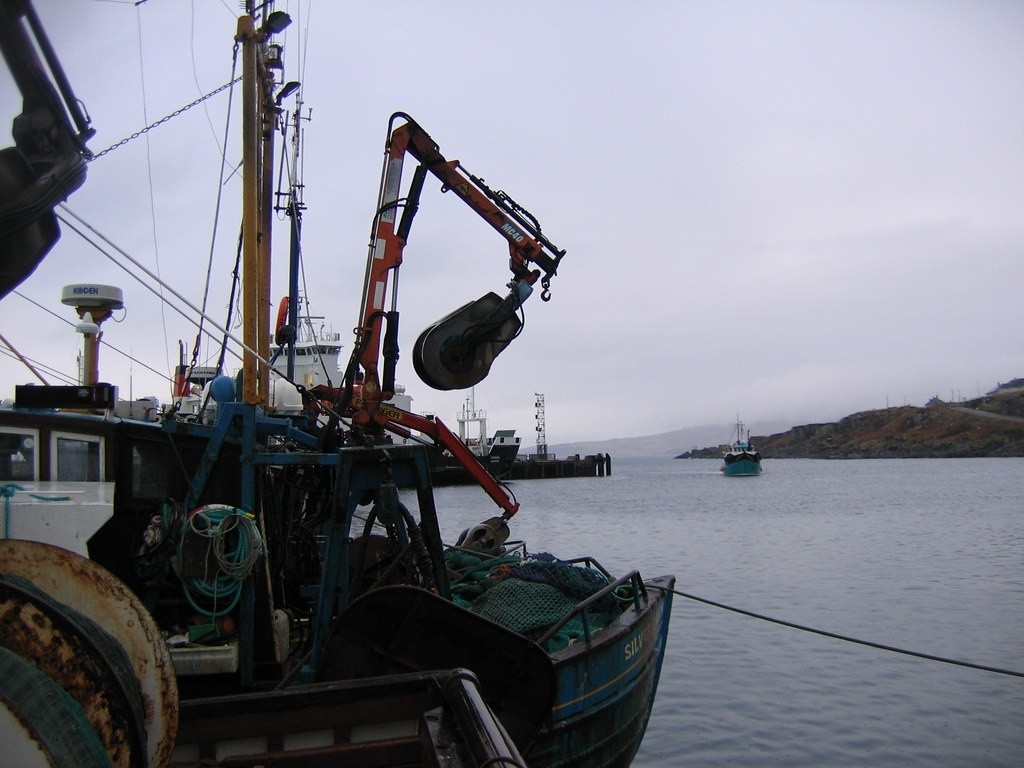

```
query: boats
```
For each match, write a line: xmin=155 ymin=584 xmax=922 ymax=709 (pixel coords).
xmin=427 ymin=387 xmax=523 ymax=486
xmin=720 ymin=411 xmax=763 ymax=477
